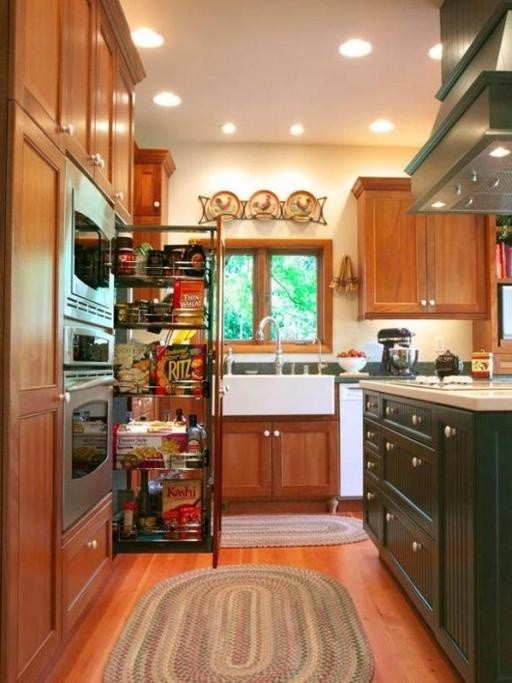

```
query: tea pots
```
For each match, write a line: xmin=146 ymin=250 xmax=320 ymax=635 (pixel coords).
xmin=435 ymin=350 xmax=463 ymax=381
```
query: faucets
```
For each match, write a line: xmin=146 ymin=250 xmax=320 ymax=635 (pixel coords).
xmin=312 ymin=336 xmax=328 ymax=375
xmin=256 ymin=316 xmax=285 ymax=375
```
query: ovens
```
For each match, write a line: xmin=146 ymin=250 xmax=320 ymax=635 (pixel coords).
xmin=64 ymin=158 xmax=118 ymax=533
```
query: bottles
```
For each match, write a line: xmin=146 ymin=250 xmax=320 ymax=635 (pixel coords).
xmin=122 ymin=490 xmax=138 ymax=537
xmin=173 ymin=408 xmax=186 ymax=424
xmin=187 ymin=414 xmax=203 ymax=467
xmin=163 ymin=410 xmax=171 ymax=423
xmin=117 ymin=248 xmax=133 ymax=273
xmin=472 ymin=351 xmax=493 ymax=378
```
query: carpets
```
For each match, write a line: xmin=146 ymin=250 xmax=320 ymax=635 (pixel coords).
xmin=100 ymin=565 xmax=375 ymax=683
xmin=213 ymin=512 xmax=372 ymax=548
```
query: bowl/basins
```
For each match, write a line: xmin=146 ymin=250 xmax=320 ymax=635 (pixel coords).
xmin=337 ymin=357 xmax=368 ymax=373
xmin=388 ymin=348 xmax=418 ymax=374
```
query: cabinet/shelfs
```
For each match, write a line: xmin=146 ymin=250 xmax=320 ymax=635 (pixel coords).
xmin=7 ymin=0 xmax=67 ymax=133
xmin=213 ymin=418 xmax=340 ymax=512
xmin=353 ymin=177 xmax=492 ymax=316
xmin=112 ymin=226 xmax=212 ymax=549
xmin=1 ymin=101 xmax=86 ymax=683
xmin=359 ymin=375 xmax=512 ymax=683
xmin=9 ymin=394 xmax=14 ymax=396
xmin=136 ymin=142 xmax=177 ymax=309
xmin=68 ymin=0 xmax=116 ymax=202
xmin=116 ymin=27 xmax=147 ymax=227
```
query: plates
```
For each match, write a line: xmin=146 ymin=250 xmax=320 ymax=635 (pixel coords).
xmin=285 ymin=191 xmax=320 ymax=222
xmin=205 ymin=191 xmax=243 ymax=220
xmin=246 ymin=190 xmax=281 ymax=220
